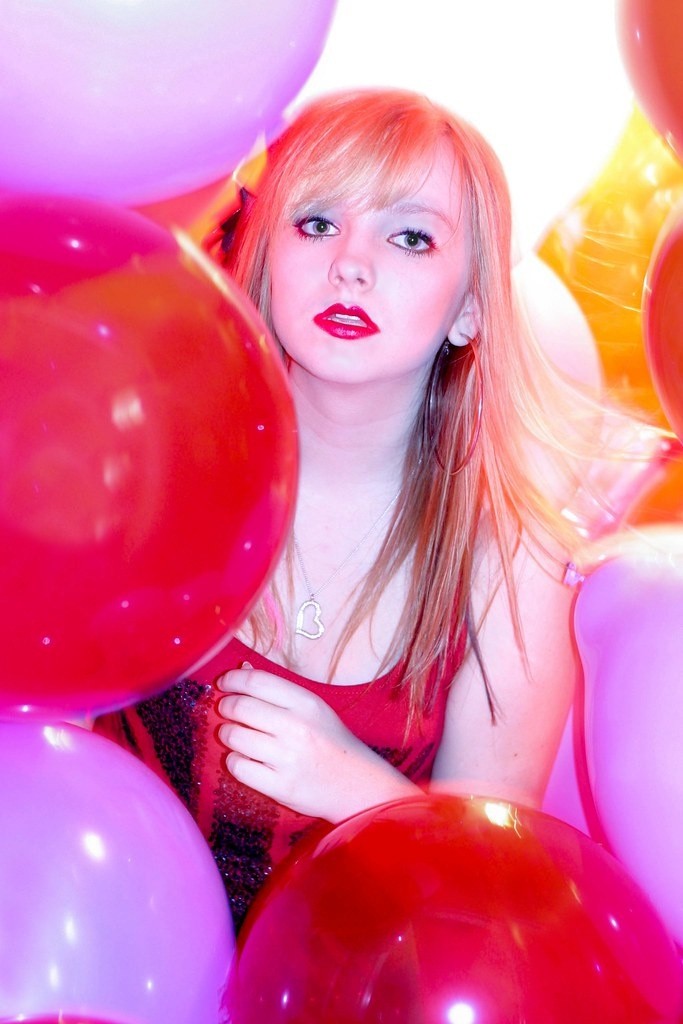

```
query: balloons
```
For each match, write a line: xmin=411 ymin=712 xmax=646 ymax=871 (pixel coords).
xmin=0 ymin=0 xmax=683 ymax=1024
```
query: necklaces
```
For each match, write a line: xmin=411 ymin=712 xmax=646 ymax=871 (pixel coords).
xmin=291 ymin=454 xmax=422 ymax=639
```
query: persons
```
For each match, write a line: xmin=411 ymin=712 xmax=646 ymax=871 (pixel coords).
xmin=70 ymin=84 xmax=580 ymax=944
